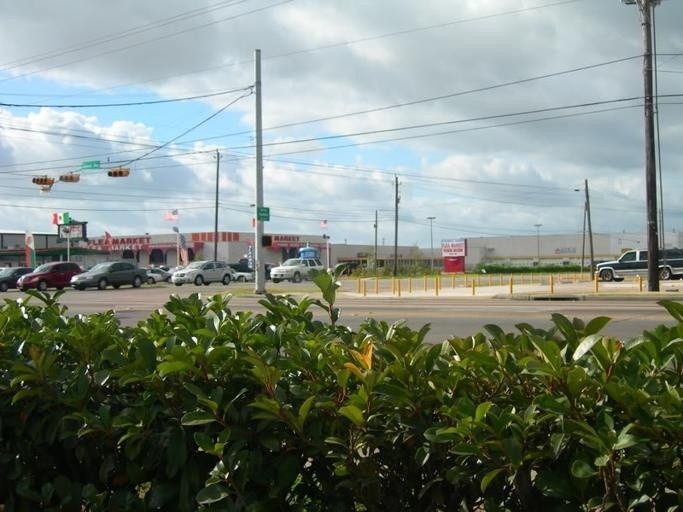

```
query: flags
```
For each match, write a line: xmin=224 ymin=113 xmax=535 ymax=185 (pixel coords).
xmin=52 ymin=212 xmax=69 ymax=224
xmin=319 ymin=219 xmax=327 ymax=228
xmin=164 ymin=210 xmax=177 ymax=220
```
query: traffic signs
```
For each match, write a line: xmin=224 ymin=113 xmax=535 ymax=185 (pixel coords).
xmin=79 ymin=161 xmax=99 ymax=170
xmin=256 ymin=207 xmax=269 ymax=221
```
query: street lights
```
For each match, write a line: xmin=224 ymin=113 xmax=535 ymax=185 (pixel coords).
xmin=574 ymin=187 xmax=594 ymax=281
xmin=426 ymin=216 xmax=436 ymax=272
xmin=533 ymin=224 xmax=543 ymax=260
xmin=172 ymin=226 xmax=180 ymax=266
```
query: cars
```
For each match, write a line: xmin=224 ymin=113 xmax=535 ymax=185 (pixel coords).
xmin=15 ymin=262 xmax=84 ymax=291
xmin=170 ymin=260 xmax=233 ymax=287
xmin=70 ymin=261 xmax=148 ymax=290
xmin=0 ymin=267 xmax=36 ymax=292
xmin=265 ymin=263 xmax=279 ymax=280
xmin=227 ymin=259 xmax=255 ymax=282
xmin=328 ymin=262 xmax=358 ymax=277
xmin=138 ymin=264 xmax=174 ymax=286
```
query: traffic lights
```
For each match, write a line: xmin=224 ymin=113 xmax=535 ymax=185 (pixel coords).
xmin=107 ymin=168 xmax=128 ymax=178
xmin=58 ymin=174 xmax=80 ymax=182
xmin=31 ymin=177 xmax=51 ymax=185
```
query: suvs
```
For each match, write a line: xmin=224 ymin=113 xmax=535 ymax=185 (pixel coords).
xmin=270 ymin=258 xmax=325 ymax=284
xmin=596 ymin=248 xmax=683 ymax=282
